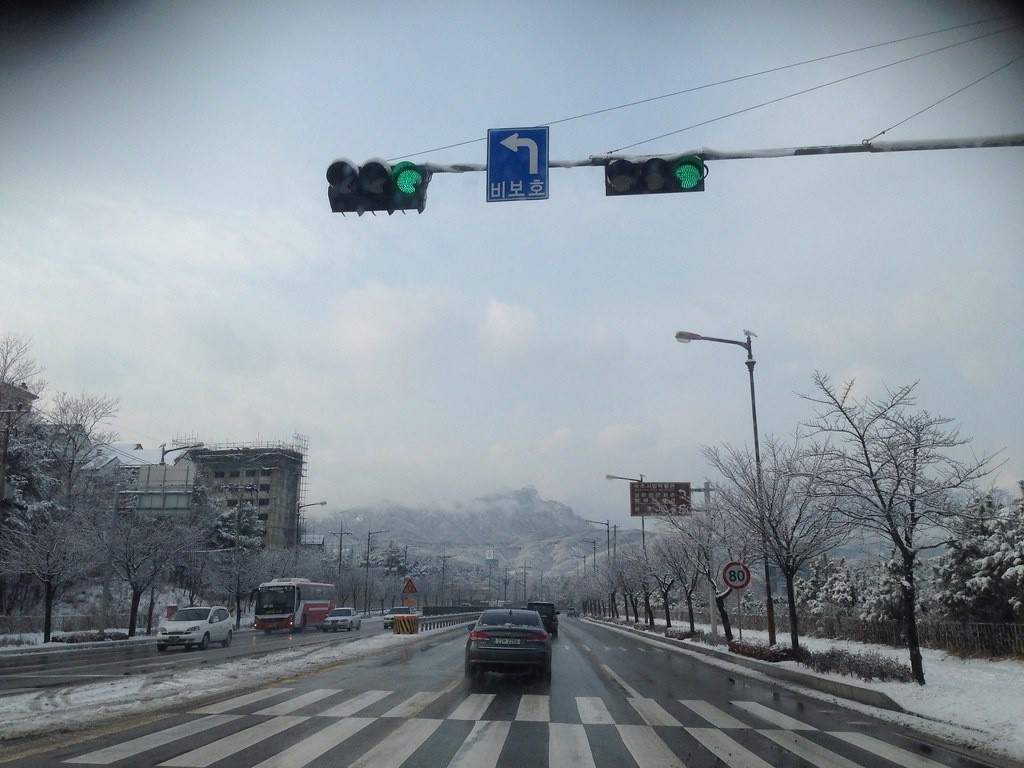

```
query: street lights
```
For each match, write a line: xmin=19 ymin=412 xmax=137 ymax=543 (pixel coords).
xmin=441 ymin=548 xmax=458 ymax=606
xmin=384 ymin=607 xmax=415 ymax=629
xmin=606 ymin=473 xmax=649 ymax=624
xmin=576 ymin=555 xmax=587 ymax=575
xmin=584 ymin=519 xmax=610 ymax=567
xmin=402 ymin=544 xmax=421 ymax=606
xmin=582 ymin=539 xmax=595 ymax=574
xmin=220 ymin=482 xmax=259 ymax=630
xmin=676 ymin=329 xmax=777 ymax=647
xmin=294 ymin=501 xmax=327 ymax=573
xmin=363 ymin=530 xmax=390 ymax=615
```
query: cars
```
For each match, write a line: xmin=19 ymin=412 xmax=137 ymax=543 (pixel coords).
xmin=464 ymin=607 xmax=553 ymax=687
xmin=323 ymin=607 xmax=361 ymax=632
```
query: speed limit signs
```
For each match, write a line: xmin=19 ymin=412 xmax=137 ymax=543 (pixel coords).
xmin=722 ymin=561 xmax=752 ymax=590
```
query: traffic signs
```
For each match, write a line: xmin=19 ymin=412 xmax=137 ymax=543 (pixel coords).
xmin=629 ymin=482 xmax=692 ymax=518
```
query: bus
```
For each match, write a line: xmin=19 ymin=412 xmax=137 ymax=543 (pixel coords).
xmin=249 ymin=578 xmax=337 ymax=635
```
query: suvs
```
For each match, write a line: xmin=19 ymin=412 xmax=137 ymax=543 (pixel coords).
xmin=155 ymin=604 xmax=233 ymax=652
xmin=521 ymin=602 xmax=560 ymax=638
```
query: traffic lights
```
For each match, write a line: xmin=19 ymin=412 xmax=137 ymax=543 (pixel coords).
xmin=604 ymin=154 xmax=706 ymax=196
xmin=325 ymin=158 xmax=432 ymax=216
